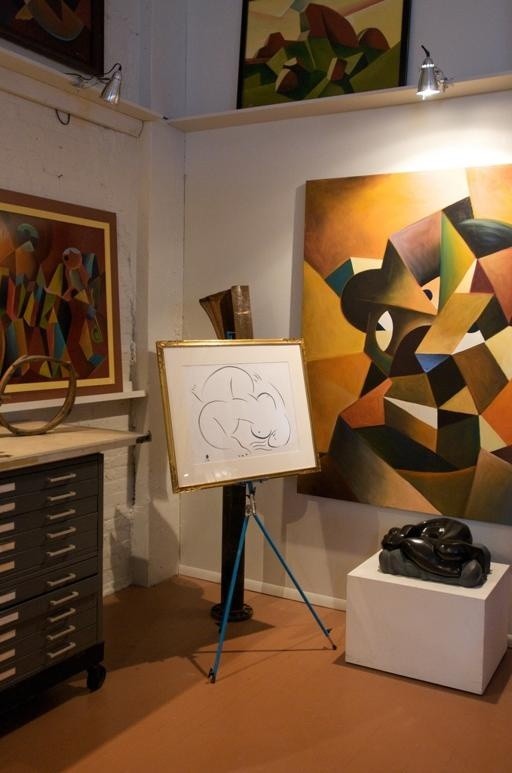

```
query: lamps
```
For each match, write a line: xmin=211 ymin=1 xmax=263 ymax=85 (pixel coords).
xmin=416 ymin=44 xmax=446 ymax=96
xmin=66 ymin=62 xmax=123 ymax=105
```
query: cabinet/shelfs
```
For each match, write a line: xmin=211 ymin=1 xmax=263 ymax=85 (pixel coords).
xmin=0 ymin=422 xmax=153 ymax=699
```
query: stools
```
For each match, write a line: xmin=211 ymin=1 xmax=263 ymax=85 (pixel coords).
xmin=345 ymin=548 xmax=511 ymax=694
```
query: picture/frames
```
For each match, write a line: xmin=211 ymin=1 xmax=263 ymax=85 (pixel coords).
xmin=156 ymin=339 xmax=322 ymax=494
xmin=236 ymin=0 xmax=412 ymax=110
xmin=0 ymin=187 xmax=123 ymax=404
xmin=0 ymin=0 xmax=105 ymax=77
